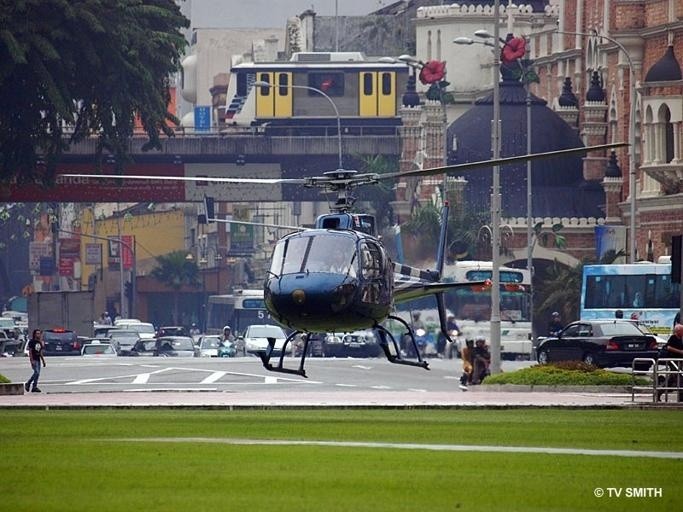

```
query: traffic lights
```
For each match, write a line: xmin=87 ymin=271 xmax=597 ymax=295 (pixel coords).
xmin=124 ymin=283 xmax=132 ymax=298
xmin=87 ymin=274 xmax=96 ymax=293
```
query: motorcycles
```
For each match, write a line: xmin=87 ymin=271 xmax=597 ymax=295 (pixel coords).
xmin=437 ymin=329 xmax=464 ymax=359
xmin=460 ymin=356 xmax=491 ymax=390
xmin=402 ymin=325 xmax=433 ymax=357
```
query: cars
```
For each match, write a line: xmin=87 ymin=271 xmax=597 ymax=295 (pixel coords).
xmin=535 ymin=320 xmax=669 ymax=374
xmin=307 ymin=330 xmax=381 ymax=358
xmin=1 ymin=317 xmax=292 ymax=358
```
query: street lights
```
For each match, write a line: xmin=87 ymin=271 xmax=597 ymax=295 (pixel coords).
xmin=186 ymin=244 xmax=222 ymax=332
xmin=247 ymin=82 xmax=343 ymax=170
xmin=380 ymin=53 xmax=451 ymax=260
xmin=453 ymin=29 xmax=535 ymax=265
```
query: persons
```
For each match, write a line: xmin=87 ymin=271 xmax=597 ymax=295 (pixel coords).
xmin=401 ymin=310 xmax=424 ymax=355
xmin=326 ymin=242 xmax=359 ymax=279
xmin=98 ymin=312 xmax=107 ymax=325
xmin=437 ymin=313 xmax=460 ymax=355
xmin=188 ymin=323 xmax=200 ymax=335
xmin=547 ymin=312 xmax=563 ymax=335
xmin=459 ymin=336 xmax=476 ymax=379
xmin=217 ymin=326 xmax=235 ymax=346
xmin=103 ymin=310 xmax=112 ymax=326
xmin=469 ymin=336 xmax=490 ymax=383
xmin=23 ymin=328 xmax=46 ymax=393
xmin=648 ymin=322 xmax=683 ymax=404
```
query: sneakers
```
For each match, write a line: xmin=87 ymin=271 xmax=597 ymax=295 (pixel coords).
xmin=25 ymin=383 xmax=30 ymax=392
xmin=31 ymin=387 xmax=41 ymax=393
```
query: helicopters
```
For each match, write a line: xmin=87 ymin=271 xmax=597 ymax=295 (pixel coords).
xmin=55 ymin=142 xmax=631 ymax=378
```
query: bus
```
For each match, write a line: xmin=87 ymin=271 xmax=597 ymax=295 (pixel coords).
xmin=580 ymin=256 xmax=683 ymax=341
xmin=2 ymin=297 xmax=28 ymax=317
xmin=381 ymin=261 xmax=532 ymax=360
xmin=206 ymin=290 xmax=279 ymax=337
xmin=226 ymin=53 xmax=411 ymax=127
